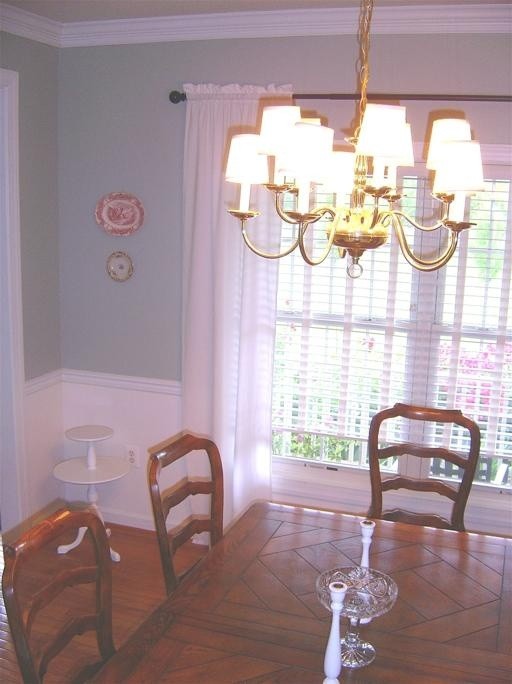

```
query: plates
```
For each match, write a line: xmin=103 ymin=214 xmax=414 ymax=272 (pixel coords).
xmin=94 ymin=192 xmax=145 ymax=237
xmin=107 ymin=250 xmax=133 ymax=282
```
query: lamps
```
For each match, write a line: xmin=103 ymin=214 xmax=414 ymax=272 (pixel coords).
xmin=223 ymin=0 xmax=485 ymax=279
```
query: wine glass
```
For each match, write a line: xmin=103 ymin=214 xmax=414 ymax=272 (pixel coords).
xmin=313 ymin=567 xmax=398 ymax=667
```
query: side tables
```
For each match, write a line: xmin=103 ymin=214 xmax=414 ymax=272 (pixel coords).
xmin=52 ymin=427 xmax=131 ymax=562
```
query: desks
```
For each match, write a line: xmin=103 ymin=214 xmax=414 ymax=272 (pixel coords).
xmin=85 ymin=500 xmax=512 ymax=684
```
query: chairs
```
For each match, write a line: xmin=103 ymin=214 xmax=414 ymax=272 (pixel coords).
xmin=4 ymin=499 xmax=116 ymax=684
xmin=147 ymin=431 xmax=224 ymax=594
xmin=365 ymin=402 xmax=481 ymax=533
